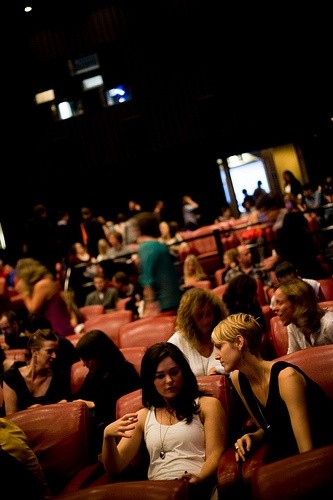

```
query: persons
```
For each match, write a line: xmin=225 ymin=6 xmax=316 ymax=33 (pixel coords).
xmin=81 ymin=206 xmax=112 ymax=259
xmin=67 ymin=329 xmax=140 ymax=466
xmin=0 ymin=417 xmax=42 ymax=470
xmin=211 ymin=313 xmax=333 ymax=462
xmin=85 ymin=221 xmax=209 ymax=319
xmin=98 ymin=216 xmax=113 ymax=230
xmin=3 ymin=329 xmax=95 ymax=416
xmin=166 ymin=288 xmax=232 ymax=377
xmin=183 ymin=197 xmax=199 ymax=230
xmin=102 ymin=341 xmax=226 ymax=500
xmin=215 ymin=169 xmax=333 ymax=355
xmin=96 ymin=200 xmax=183 ymax=261
xmin=54 ymin=209 xmax=78 ymax=263
xmin=21 ymin=204 xmax=56 ymax=281
xmin=0 ymin=256 xmax=77 ymax=338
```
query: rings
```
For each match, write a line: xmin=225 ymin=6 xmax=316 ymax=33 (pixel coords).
xmin=234 ymin=447 xmax=238 ymax=452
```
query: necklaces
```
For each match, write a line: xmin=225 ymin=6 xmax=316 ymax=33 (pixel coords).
xmin=198 ymin=345 xmax=211 ymax=376
xmin=253 ymin=361 xmax=270 ymax=428
xmin=160 ymin=407 xmax=174 ymax=459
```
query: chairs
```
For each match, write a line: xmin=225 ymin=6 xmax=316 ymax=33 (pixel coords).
xmin=0 ymin=215 xmax=333 ymax=500
